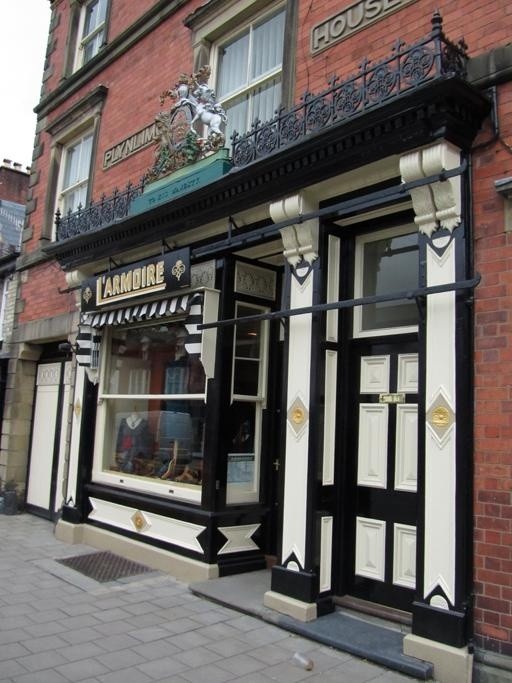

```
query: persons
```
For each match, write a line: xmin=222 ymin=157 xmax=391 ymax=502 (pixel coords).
xmin=115 ymin=416 xmax=149 ymax=473
xmin=154 ymin=339 xmax=206 ymax=443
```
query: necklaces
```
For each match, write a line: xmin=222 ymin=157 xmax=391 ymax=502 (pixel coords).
xmin=129 ymin=416 xmax=141 ymax=428
